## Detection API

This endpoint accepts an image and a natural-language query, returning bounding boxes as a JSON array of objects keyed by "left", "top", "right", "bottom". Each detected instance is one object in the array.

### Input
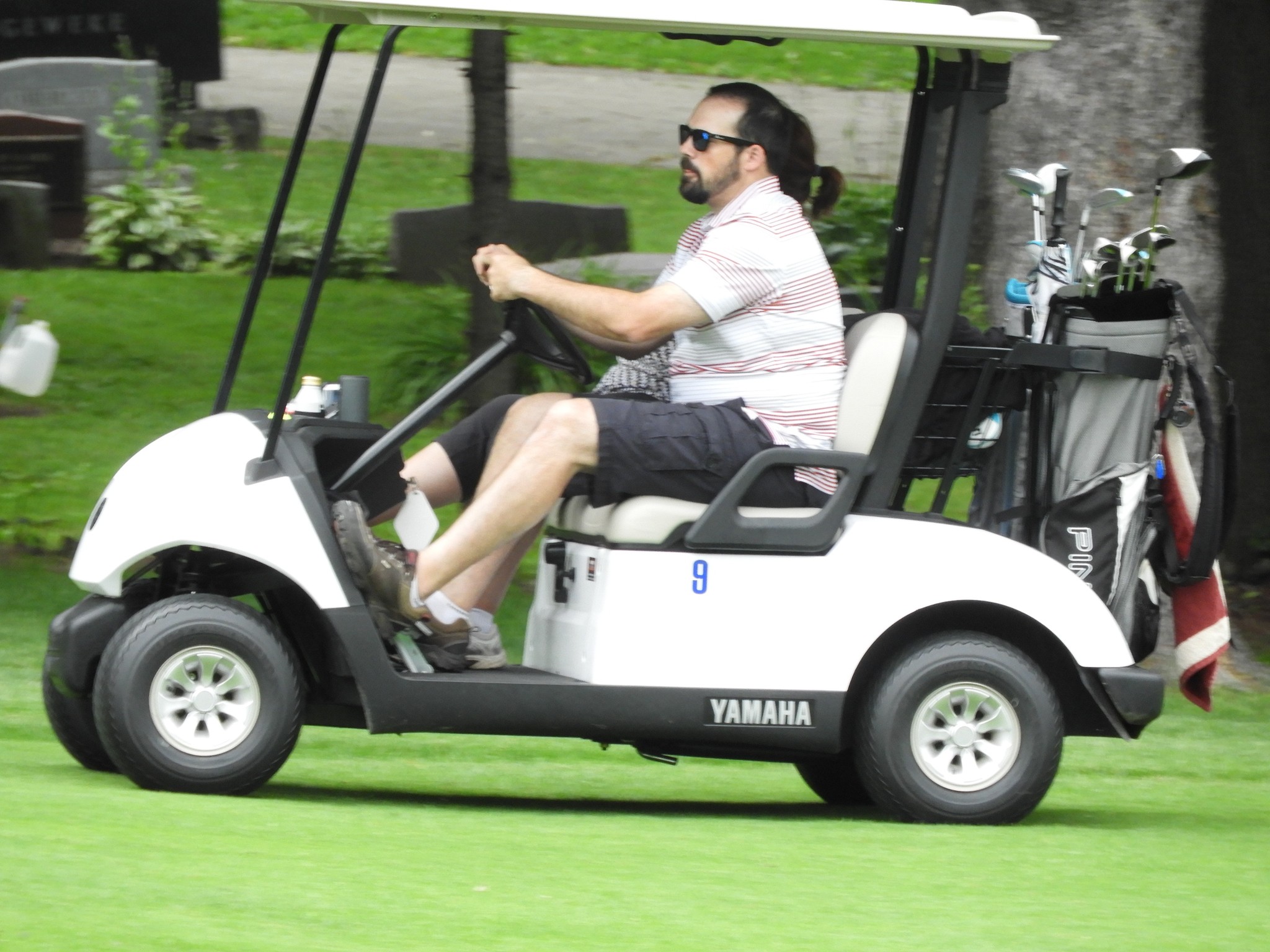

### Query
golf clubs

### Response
[{"left": 1001, "top": 148, "right": 1212, "bottom": 294}]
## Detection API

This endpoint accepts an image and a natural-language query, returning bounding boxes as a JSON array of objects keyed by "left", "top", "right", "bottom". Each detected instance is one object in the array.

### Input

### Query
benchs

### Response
[{"left": 542, "top": 306, "right": 920, "bottom": 559}]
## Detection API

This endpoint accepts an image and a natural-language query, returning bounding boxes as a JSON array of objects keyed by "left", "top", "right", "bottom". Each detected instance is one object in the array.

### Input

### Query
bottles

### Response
[
  {"left": 294, "top": 376, "right": 324, "bottom": 419},
  {"left": 0, "top": 320, "right": 60, "bottom": 398}
]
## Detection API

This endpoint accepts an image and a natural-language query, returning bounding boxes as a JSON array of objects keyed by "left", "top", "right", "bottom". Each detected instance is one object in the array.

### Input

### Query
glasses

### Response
[{"left": 679, "top": 123, "right": 763, "bottom": 153}]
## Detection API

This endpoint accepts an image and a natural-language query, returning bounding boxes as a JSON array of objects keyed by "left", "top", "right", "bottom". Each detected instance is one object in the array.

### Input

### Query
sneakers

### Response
[
  {"left": 332, "top": 500, "right": 430, "bottom": 642},
  {"left": 450, "top": 608, "right": 506, "bottom": 670},
  {"left": 407, "top": 608, "right": 471, "bottom": 673}
]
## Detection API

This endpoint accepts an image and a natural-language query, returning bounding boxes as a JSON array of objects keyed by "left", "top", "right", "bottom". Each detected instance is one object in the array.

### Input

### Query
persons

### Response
[{"left": 331, "top": 81, "right": 848, "bottom": 674}]
